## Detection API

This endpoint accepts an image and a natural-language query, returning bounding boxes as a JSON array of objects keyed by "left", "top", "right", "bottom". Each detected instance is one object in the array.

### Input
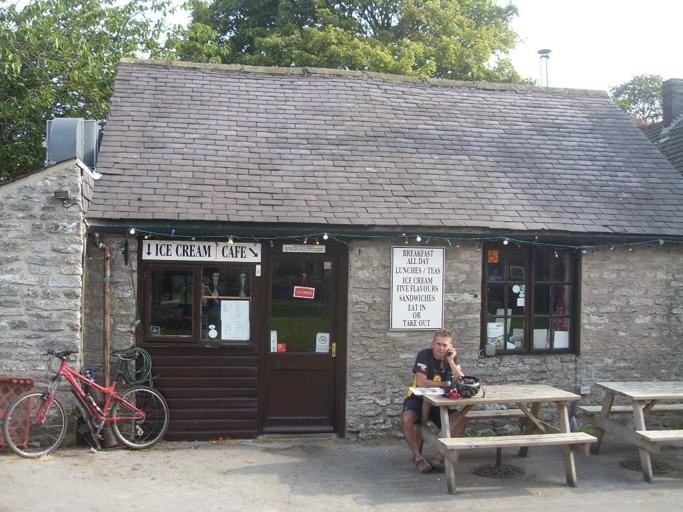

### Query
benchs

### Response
[{"left": 412, "top": 379, "right": 683, "bottom": 495}]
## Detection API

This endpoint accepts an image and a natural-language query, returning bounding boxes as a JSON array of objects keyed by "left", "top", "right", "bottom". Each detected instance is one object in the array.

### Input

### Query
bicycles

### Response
[{"left": 1, "top": 348, "right": 169, "bottom": 459}]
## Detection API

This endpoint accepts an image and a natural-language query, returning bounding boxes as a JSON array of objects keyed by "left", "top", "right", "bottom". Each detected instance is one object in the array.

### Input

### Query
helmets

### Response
[{"left": 456, "top": 375, "right": 481, "bottom": 397}]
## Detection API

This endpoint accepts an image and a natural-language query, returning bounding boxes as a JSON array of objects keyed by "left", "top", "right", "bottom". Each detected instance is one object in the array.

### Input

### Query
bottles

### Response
[{"left": 446, "top": 373, "right": 454, "bottom": 393}]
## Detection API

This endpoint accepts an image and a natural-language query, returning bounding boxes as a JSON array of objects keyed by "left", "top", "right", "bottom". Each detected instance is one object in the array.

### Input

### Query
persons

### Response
[
  {"left": 401, "top": 330, "right": 467, "bottom": 474},
  {"left": 201, "top": 280, "right": 218, "bottom": 338},
  {"left": 157, "top": 292, "right": 170, "bottom": 311}
]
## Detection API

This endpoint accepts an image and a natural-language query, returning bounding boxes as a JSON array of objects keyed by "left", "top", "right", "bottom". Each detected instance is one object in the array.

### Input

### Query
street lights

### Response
[{"left": 537, "top": 48, "right": 551, "bottom": 87}]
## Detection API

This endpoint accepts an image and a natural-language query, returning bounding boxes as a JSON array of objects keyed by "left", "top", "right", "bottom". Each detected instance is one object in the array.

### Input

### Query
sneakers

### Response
[{"left": 415, "top": 454, "right": 444, "bottom": 474}]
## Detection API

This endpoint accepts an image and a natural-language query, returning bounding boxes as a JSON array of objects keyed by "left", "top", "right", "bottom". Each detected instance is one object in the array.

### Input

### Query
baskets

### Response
[{"left": 0, "top": 375, "right": 36, "bottom": 447}]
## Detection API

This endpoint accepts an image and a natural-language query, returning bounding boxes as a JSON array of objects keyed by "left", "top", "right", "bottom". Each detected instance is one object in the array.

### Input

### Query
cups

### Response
[{"left": 484, "top": 343, "right": 495, "bottom": 356}]
